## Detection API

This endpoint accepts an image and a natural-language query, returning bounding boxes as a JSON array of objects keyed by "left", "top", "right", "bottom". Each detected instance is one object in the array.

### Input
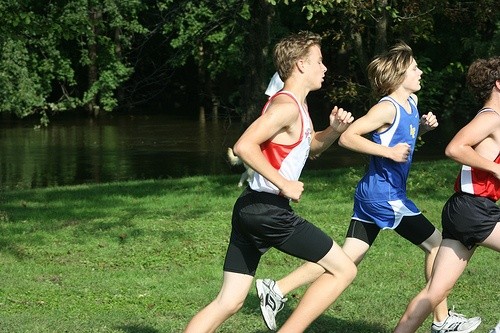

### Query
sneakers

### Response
[
  {"left": 431, "top": 310, "right": 482, "bottom": 333},
  {"left": 256, "top": 279, "right": 288, "bottom": 331}
]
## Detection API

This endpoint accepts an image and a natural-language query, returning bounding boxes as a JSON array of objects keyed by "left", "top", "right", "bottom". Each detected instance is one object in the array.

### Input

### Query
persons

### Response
[
  {"left": 184, "top": 30, "right": 355, "bottom": 333},
  {"left": 392, "top": 57, "right": 500, "bottom": 333},
  {"left": 255, "top": 40, "right": 482, "bottom": 333}
]
